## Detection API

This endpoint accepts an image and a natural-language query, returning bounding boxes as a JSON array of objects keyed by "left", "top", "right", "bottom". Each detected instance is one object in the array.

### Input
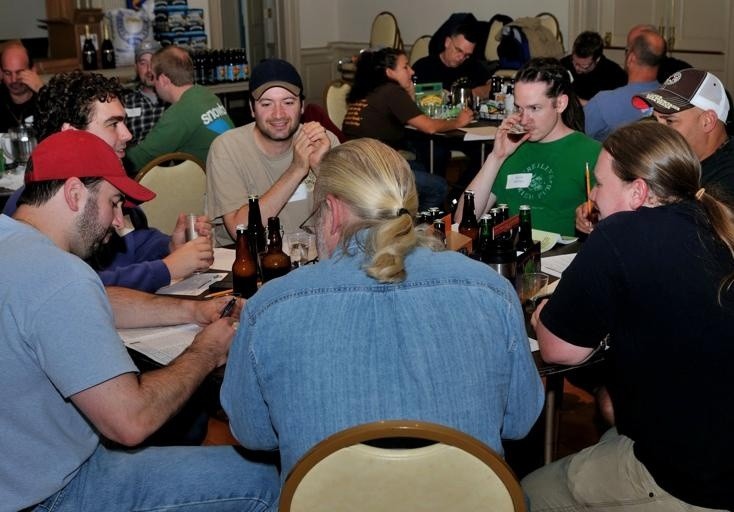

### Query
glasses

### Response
[
  {"left": 298, "top": 205, "right": 323, "bottom": 235},
  {"left": 571, "top": 60, "right": 594, "bottom": 71},
  {"left": 449, "top": 36, "right": 473, "bottom": 61}
]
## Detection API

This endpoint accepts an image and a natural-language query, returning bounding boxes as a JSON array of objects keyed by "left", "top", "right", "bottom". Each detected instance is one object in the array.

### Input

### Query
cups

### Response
[
  {"left": 522, "top": 273, "right": 549, "bottom": 305},
  {"left": 507, "top": 113, "right": 536, "bottom": 143},
  {"left": 286, "top": 233, "right": 311, "bottom": 270}
]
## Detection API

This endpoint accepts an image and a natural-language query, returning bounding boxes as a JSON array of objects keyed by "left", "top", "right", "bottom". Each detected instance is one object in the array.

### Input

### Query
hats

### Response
[
  {"left": 22, "top": 129, "right": 157, "bottom": 208},
  {"left": 248, "top": 57, "right": 304, "bottom": 102},
  {"left": 630, "top": 68, "right": 729, "bottom": 127},
  {"left": 133, "top": 37, "right": 161, "bottom": 64}
]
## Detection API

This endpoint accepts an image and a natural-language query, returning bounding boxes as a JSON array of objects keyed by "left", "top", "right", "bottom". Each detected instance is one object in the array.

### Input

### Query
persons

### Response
[
  {"left": 0, "top": 130, "right": 280, "bottom": 511},
  {"left": 631, "top": 68, "right": 734, "bottom": 216},
  {"left": 519, "top": 121, "right": 734, "bottom": 512},
  {"left": 124, "top": 47, "right": 236, "bottom": 172},
  {"left": 118, "top": 40, "right": 172, "bottom": 152},
  {"left": 454, "top": 56, "right": 603, "bottom": 239},
  {"left": 0, "top": 39, "right": 54, "bottom": 131},
  {"left": 411, "top": 24, "right": 496, "bottom": 105},
  {"left": 625, "top": 25, "right": 694, "bottom": 83},
  {"left": 221, "top": 139, "right": 545, "bottom": 504},
  {"left": 1, "top": 71, "right": 215, "bottom": 288},
  {"left": 559, "top": 31, "right": 628, "bottom": 106},
  {"left": 342, "top": 47, "right": 474, "bottom": 206},
  {"left": 584, "top": 30, "right": 694, "bottom": 153},
  {"left": 205, "top": 58, "right": 341, "bottom": 252}
]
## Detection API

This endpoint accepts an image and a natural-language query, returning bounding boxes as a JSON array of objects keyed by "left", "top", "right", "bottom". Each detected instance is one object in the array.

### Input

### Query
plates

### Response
[{"left": 479, "top": 110, "right": 507, "bottom": 120}]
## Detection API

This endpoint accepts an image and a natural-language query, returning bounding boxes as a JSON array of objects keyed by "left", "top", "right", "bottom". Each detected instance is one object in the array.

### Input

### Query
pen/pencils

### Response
[
  {"left": 205, "top": 282, "right": 263, "bottom": 298},
  {"left": 465, "top": 99, "right": 467, "bottom": 113},
  {"left": 586, "top": 162, "right": 591, "bottom": 214},
  {"left": 219, "top": 297, "right": 237, "bottom": 319}
]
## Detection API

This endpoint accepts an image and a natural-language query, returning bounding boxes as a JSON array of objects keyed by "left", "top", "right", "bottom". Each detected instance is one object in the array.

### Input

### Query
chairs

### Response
[
  {"left": 278, "top": 421, "right": 525, "bottom": 507},
  {"left": 130, "top": 152, "right": 212, "bottom": 244}
]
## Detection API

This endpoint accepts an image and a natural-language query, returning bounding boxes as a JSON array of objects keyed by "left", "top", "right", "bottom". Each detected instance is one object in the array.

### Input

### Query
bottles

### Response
[
  {"left": 232, "top": 223, "right": 257, "bottom": 299},
  {"left": 506, "top": 84, "right": 512, "bottom": 94},
  {"left": 261, "top": 217, "right": 291, "bottom": 284},
  {"left": 82, "top": 25, "right": 97, "bottom": 71},
  {"left": 187, "top": 48, "right": 249, "bottom": 86},
  {"left": 476, "top": 213, "right": 496, "bottom": 264},
  {"left": 245, "top": 194, "right": 265, "bottom": 262},
  {"left": 432, "top": 217, "right": 447, "bottom": 248},
  {"left": 101, "top": 23, "right": 116, "bottom": 69},
  {"left": 515, "top": 204, "right": 535, "bottom": 258},
  {"left": 458, "top": 190, "right": 479, "bottom": 244},
  {"left": 498, "top": 203, "right": 513, "bottom": 260},
  {"left": 489, "top": 75, "right": 497, "bottom": 100},
  {"left": 489, "top": 208, "right": 505, "bottom": 262}
]
